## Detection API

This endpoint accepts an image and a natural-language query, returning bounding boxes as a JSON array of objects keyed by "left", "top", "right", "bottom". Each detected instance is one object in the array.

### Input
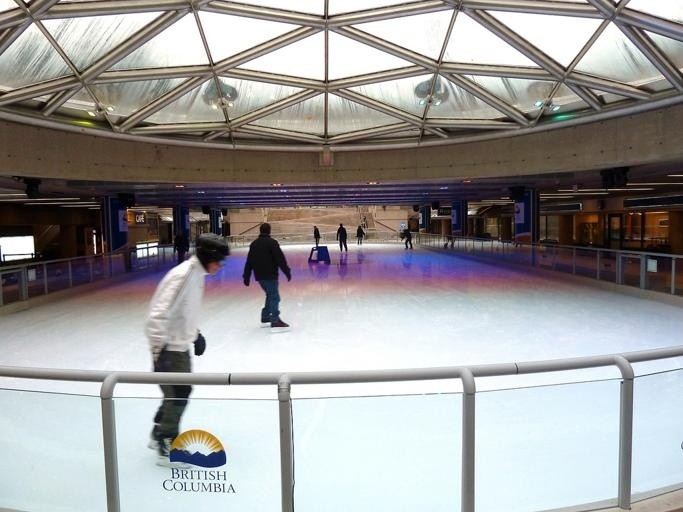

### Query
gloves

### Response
[
  {"left": 194, "top": 334, "right": 206, "bottom": 356},
  {"left": 285, "top": 273, "right": 292, "bottom": 282},
  {"left": 244, "top": 278, "right": 250, "bottom": 286}
]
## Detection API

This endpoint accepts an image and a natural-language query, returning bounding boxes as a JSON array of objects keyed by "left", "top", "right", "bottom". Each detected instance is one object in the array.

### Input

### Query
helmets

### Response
[{"left": 195, "top": 232, "right": 230, "bottom": 263}]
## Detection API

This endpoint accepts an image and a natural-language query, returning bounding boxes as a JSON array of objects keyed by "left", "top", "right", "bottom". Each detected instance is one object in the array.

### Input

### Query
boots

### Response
[
  {"left": 151, "top": 416, "right": 173, "bottom": 457},
  {"left": 271, "top": 319, "right": 289, "bottom": 327},
  {"left": 261, "top": 316, "right": 273, "bottom": 322}
]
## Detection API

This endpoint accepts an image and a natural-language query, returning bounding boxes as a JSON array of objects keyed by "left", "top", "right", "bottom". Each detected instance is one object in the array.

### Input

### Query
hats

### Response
[{"left": 261, "top": 223, "right": 271, "bottom": 234}]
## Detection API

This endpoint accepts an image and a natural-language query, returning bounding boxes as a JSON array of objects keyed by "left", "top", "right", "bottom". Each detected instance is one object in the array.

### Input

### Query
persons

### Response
[
  {"left": 336, "top": 223, "right": 347, "bottom": 252},
  {"left": 144, "top": 233, "right": 229, "bottom": 469},
  {"left": 401, "top": 229, "right": 412, "bottom": 249},
  {"left": 314, "top": 226, "right": 320, "bottom": 246},
  {"left": 174, "top": 229, "right": 189, "bottom": 264},
  {"left": 357, "top": 226, "right": 365, "bottom": 245},
  {"left": 243, "top": 223, "right": 291, "bottom": 327}
]
